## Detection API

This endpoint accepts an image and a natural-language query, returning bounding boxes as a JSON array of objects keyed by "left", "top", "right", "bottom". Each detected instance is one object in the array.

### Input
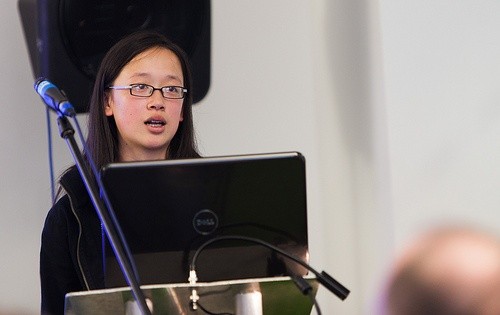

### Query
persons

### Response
[{"left": 40, "top": 31, "right": 310, "bottom": 315}]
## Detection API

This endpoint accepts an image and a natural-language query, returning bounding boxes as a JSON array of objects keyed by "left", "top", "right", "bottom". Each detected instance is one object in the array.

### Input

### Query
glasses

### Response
[{"left": 108, "top": 84, "right": 188, "bottom": 99}]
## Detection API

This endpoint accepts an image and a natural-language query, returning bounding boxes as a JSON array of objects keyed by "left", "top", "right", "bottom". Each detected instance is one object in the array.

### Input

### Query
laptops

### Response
[{"left": 98, "top": 151, "right": 310, "bottom": 288}]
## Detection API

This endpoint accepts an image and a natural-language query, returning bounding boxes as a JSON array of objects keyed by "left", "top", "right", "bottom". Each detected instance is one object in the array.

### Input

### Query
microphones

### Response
[
  {"left": 188, "top": 234, "right": 351, "bottom": 301},
  {"left": 293, "top": 275, "right": 321, "bottom": 315},
  {"left": 33, "top": 77, "right": 76, "bottom": 118}
]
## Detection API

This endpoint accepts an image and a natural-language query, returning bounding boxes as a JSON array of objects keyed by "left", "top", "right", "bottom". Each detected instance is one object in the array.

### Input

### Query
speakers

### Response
[{"left": 17, "top": 0, "right": 211, "bottom": 113}]
